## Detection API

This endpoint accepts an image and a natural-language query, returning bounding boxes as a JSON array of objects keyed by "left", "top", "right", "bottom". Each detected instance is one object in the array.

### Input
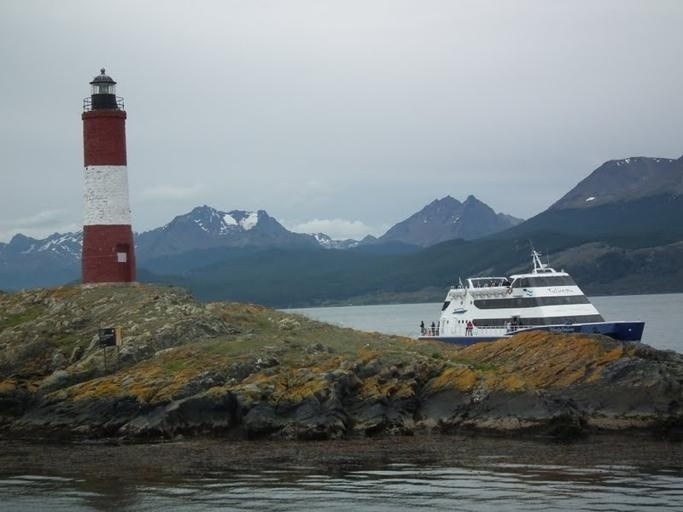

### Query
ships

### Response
[{"left": 418, "top": 234, "right": 645, "bottom": 347}]
